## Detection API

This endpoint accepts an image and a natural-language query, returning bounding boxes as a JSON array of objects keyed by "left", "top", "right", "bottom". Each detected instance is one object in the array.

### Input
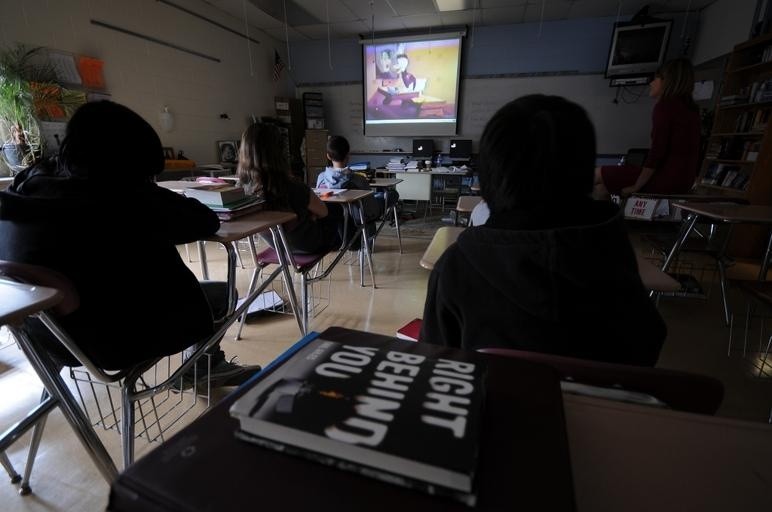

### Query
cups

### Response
[{"left": 425, "top": 161, "right": 431, "bottom": 171}]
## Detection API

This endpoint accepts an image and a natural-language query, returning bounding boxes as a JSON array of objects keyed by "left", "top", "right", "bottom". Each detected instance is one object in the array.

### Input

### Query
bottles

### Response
[
  {"left": 435, "top": 154, "right": 443, "bottom": 167},
  {"left": 440, "top": 217, "right": 454, "bottom": 224}
]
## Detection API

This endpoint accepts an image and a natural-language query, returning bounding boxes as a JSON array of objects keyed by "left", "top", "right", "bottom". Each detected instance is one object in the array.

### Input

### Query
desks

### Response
[
  {"left": 1, "top": 278, "right": 120, "bottom": 498},
  {"left": 98, "top": 323, "right": 770, "bottom": 512},
  {"left": 152, "top": 153, "right": 771, "bottom": 388}
]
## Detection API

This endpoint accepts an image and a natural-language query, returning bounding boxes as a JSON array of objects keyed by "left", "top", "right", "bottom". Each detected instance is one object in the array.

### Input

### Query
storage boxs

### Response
[{"left": 273, "top": 90, "right": 329, "bottom": 186}]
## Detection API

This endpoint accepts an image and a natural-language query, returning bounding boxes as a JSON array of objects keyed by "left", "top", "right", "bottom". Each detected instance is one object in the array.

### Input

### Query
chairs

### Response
[
  {"left": 468, "top": 337, "right": 728, "bottom": 421},
  {"left": 0, "top": 190, "right": 243, "bottom": 496}
]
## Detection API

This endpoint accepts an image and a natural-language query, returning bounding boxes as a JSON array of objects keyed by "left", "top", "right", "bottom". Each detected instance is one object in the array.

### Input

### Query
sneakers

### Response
[{"left": 169, "top": 355, "right": 261, "bottom": 394}]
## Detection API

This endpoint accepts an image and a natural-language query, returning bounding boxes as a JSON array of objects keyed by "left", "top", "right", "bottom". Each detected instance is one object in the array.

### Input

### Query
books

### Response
[
  {"left": 385, "top": 158, "right": 423, "bottom": 172},
  {"left": 229, "top": 338, "right": 489, "bottom": 508},
  {"left": 702, "top": 44, "right": 772, "bottom": 191},
  {"left": 155, "top": 180, "right": 267, "bottom": 222}
]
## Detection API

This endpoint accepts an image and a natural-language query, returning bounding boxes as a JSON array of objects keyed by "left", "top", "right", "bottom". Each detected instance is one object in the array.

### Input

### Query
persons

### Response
[
  {"left": 7, "top": 99, "right": 262, "bottom": 394},
  {"left": 590, "top": 58, "right": 705, "bottom": 200},
  {"left": 419, "top": 94, "right": 726, "bottom": 417},
  {"left": 234, "top": 122, "right": 361, "bottom": 256},
  {"left": 315, "top": 136, "right": 399, "bottom": 249}
]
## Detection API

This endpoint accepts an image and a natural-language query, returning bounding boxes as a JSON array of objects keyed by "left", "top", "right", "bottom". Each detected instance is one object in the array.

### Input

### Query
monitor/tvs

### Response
[{"left": 604, "top": 19, "right": 674, "bottom": 78}]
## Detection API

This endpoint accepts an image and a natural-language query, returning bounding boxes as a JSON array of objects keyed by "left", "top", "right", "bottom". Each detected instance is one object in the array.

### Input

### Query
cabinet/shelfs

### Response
[
  {"left": 38, "top": 82, "right": 112, "bottom": 131},
  {"left": 674, "top": 31, "right": 772, "bottom": 264}
]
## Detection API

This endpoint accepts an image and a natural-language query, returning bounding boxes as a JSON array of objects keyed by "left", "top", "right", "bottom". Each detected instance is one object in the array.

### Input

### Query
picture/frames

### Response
[{"left": 214, "top": 139, "right": 239, "bottom": 165}]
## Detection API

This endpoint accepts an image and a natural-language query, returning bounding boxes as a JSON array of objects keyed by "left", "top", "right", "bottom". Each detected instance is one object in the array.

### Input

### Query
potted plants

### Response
[{"left": 1, "top": 37, "right": 100, "bottom": 179}]
